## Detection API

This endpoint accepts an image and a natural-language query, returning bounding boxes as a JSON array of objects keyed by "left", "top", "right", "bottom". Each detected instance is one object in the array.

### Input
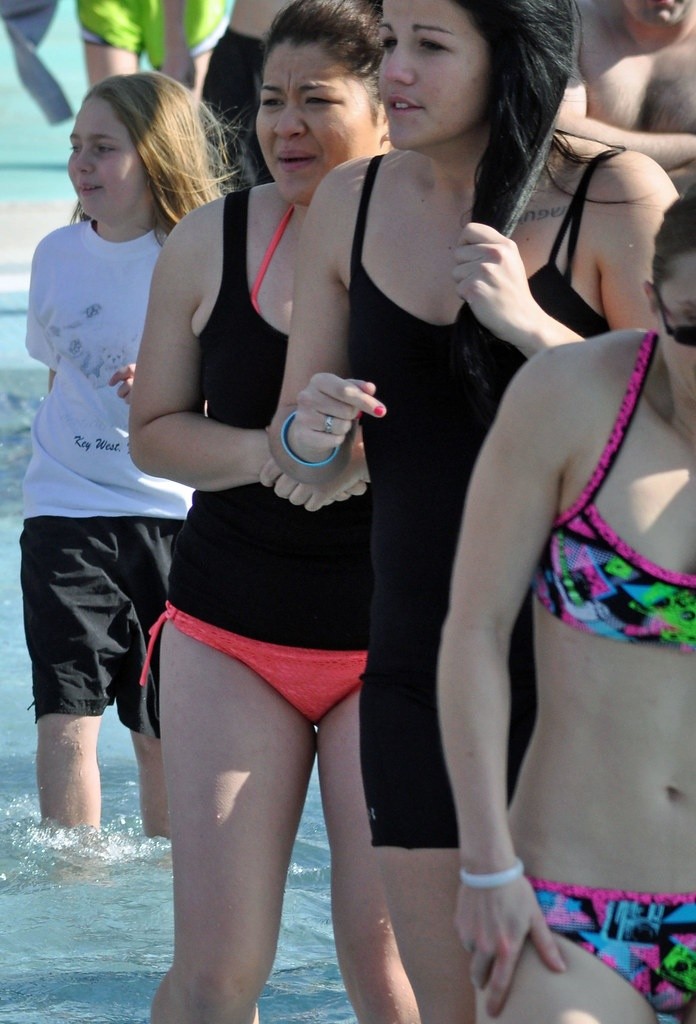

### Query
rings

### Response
[{"left": 324, "top": 415, "right": 334, "bottom": 433}]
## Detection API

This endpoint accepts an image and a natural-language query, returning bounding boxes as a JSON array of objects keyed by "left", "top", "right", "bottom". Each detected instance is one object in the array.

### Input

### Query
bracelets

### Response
[
  {"left": 458, "top": 859, "right": 524, "bottom": 888},
  {"left": 283, "top": 411, "right": 341, "bottom": 468}
]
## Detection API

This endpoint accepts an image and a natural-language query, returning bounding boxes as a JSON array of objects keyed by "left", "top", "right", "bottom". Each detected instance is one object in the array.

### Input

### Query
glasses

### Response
[{"left": 653, "top": 284, "right": 696, "bottom": 347}]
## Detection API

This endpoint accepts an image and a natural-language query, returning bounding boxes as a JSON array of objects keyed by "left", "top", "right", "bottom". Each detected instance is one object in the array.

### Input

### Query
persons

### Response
[{"left": 0, "top": 0, "right": 696, "bottom": 1024}]
